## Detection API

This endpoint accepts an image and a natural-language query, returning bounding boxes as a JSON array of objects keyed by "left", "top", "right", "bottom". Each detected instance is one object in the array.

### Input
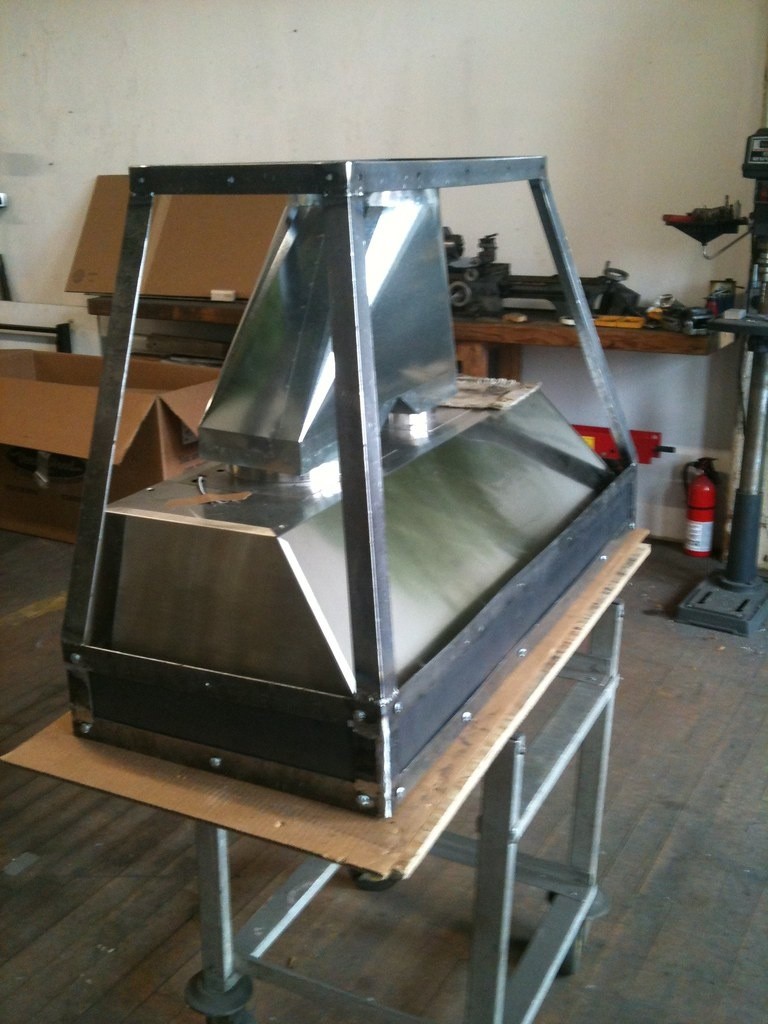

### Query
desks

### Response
[
  {"left": 88, "top": 295, "right": 746, "bottom": 381},
  {"left": 0, "top": 525, "right": 651, "bottom": 1023}
]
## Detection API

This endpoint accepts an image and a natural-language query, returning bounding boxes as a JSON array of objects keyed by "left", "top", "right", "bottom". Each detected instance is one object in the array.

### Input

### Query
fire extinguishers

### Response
[{"left": 680, "top": 457, "right": 720, "bottom": 557}]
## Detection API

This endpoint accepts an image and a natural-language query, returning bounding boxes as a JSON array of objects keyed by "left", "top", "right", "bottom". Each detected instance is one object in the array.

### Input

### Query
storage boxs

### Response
[
  {"left": 64, "top": 173, "right": 290, "bottom": 299},
  {"left": 0, "top": 348, "right": 225, "bottom": 542}
]
